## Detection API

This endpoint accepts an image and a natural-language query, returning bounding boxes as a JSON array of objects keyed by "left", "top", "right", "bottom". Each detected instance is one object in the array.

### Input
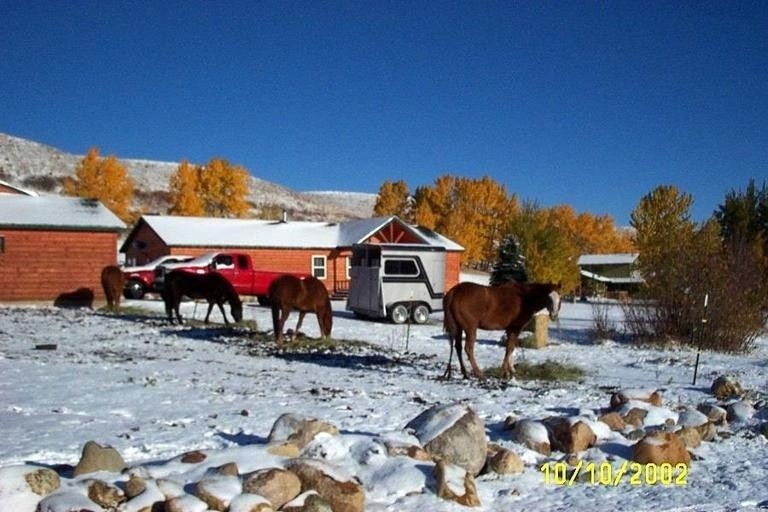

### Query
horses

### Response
[
  {"left": 159, "top": 269, "right": 244, "bottom": 327},
  {"left": 270, "top": 274, "right": 333, "bottom": 345},
  {"left": 441, "top": 279, "right": 563, "bottom": 381},
  {"left": 101, "top": 266, "right": 127, "bottom": 310}
]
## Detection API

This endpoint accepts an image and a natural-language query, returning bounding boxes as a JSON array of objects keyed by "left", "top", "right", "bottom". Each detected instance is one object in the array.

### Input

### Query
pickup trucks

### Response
[
  {"left": 119, "top": 252, "right": 197, "bottom": 301},
  {"left": 154, "top": 247, "right": 313, "bottom": 308}
]
